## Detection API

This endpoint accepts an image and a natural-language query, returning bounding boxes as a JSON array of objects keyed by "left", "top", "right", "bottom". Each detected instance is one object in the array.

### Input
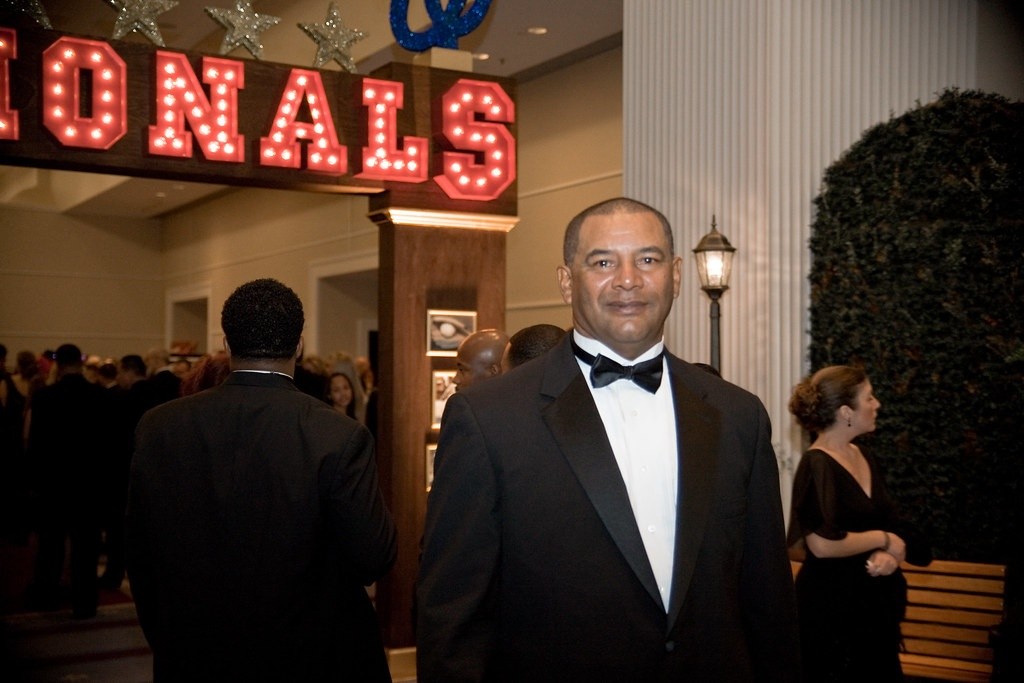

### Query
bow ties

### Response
[{"left": 570, "top": 329, "right": 665, "bottom": 395}]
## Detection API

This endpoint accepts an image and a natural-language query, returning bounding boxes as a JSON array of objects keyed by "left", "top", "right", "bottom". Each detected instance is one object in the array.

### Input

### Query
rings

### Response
[{"left": 878, "top": 568, "right": 882, "bottom": 572}]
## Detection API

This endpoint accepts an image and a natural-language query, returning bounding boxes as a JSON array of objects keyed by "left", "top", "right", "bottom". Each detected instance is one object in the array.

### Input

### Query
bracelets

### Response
[{"left": 882, "top": 530, "right": 891, "bottom": 552}]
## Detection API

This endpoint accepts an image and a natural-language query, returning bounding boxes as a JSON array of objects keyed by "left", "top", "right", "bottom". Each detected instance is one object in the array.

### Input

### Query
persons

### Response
[
  {"left": 0, "top": 341, "right": 377, "bottom": 619},
  {"left": 504, "top": 324, "right": 566, "bottom": 375},
  {"left": 415, "top": 198, "right": 807, "bottom": 683},
  {"left": 451, "top": 328, "right": 510, "bottom": 392},
  {"left": 125, "top": 278, "right": 397, "bottom": 683},
  {"left": 782, "top": 365, "right": 910, "bottom": 683}
]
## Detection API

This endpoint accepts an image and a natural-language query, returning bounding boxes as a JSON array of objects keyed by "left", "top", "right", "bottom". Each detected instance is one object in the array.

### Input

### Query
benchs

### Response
[{"left": 784, "top": 555, "right": 1006, "bottom": 682}]
